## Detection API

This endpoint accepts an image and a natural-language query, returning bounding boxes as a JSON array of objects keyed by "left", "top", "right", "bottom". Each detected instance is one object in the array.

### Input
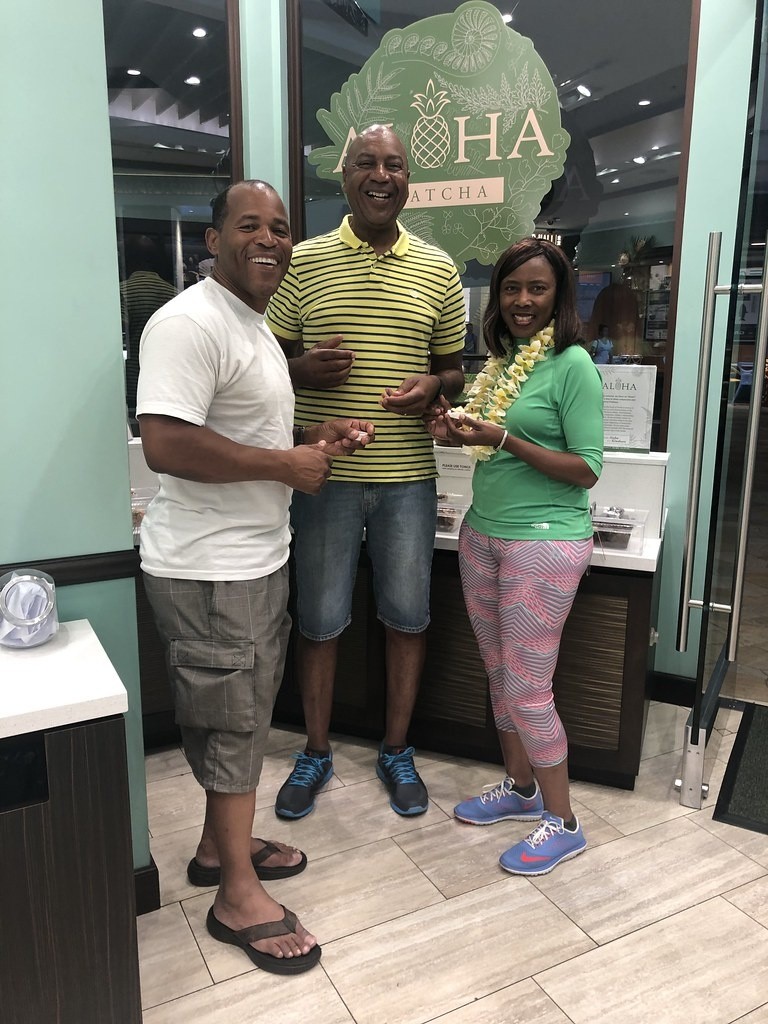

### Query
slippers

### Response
[
  {"left": 205, "top": 903, "right": 322, "bottom": 975},
  {"left": 188, "top": 838, "right": 308, "bottom": 887}
]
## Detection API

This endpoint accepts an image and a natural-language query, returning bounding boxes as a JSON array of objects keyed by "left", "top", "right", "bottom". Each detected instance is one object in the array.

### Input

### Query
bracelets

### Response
[
  {"left": 493, "top": 429, "right": 508, "bottom": 452},
  {"left": 293, "top": 427, "right": 305, "bottom": 447},
  {"left": 432, "top": 378, "right": 443, "bottom": 404}
]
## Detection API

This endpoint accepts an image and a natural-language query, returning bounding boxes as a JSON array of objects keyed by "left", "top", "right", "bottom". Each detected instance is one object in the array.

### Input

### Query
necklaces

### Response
[{"left": 448, "top": 317, "right": 554, "bottom": 463}]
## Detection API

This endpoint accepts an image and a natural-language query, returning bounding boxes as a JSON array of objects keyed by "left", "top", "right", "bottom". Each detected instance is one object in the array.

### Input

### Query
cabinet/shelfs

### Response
[
  {"left": 267, "top": 539, "right": 661, "bottom": 790},
  {"left": 0, "top": 618, "right": 144, "bottom": 1024}
]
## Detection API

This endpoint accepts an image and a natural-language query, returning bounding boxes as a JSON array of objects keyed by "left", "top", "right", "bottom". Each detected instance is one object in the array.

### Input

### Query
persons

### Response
[
  {"left": 135, "top": 179, "right": 375, "bottom": 975},
  {"left": 589, "top": 325, "right": 614, "bottom": 364},
  {"left": 586, "top": 283, "right": 658, "bottom": 365},
  {"left": 428, "top": 237, "right": 604, "bottom": 876},
  {"left": 263, "top": 124, "right": 466, "bottom": 820},
  {"left": 463, "top": 323, "right": 477, "bottom": 373},
  {"left": 120, "top": 235, "right": 181, "bottom": 437}
]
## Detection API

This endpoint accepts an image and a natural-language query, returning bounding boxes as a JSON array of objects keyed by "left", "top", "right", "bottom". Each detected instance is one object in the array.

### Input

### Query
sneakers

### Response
[
  {"left": 498, "top": 810, "right": 587, "bottom": 876},
  {"left": 275, "top": 742, "right": 334, "bottom": 817},
  {"left": 376, "top": 738, "right": 429, "bottom": 814},
  {"left": 454, "top": 766, "right": 544, "bottom": 825}
]
